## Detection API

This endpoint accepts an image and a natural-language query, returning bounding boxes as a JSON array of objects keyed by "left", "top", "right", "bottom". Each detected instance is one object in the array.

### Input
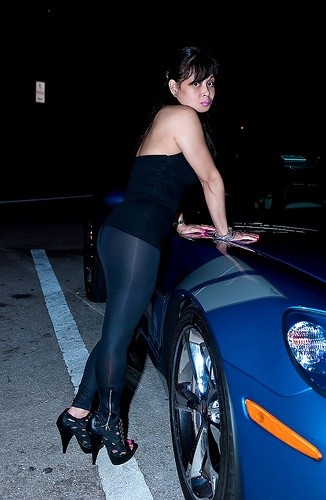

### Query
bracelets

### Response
[
  {"left": 175, "top": 219, "right": 184, "bottom": 226},
  {"left": 215, "top": 227, "right": 236, "bottom": 241}
]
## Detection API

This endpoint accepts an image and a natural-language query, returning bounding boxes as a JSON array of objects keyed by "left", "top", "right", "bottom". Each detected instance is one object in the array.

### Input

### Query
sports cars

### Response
[{"left": 81, "top": 190, "right": 324, "bottom": 499}]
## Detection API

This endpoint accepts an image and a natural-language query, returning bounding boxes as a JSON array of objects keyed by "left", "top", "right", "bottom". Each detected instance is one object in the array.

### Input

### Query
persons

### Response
[{"left": 57, "top": 50, "right": 259, "bottom": 472}]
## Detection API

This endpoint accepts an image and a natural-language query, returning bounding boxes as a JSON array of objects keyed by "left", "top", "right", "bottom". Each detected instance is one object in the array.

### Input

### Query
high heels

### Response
[{"left": 56, "top": 408, "right": 138, "bottom": 465}]
